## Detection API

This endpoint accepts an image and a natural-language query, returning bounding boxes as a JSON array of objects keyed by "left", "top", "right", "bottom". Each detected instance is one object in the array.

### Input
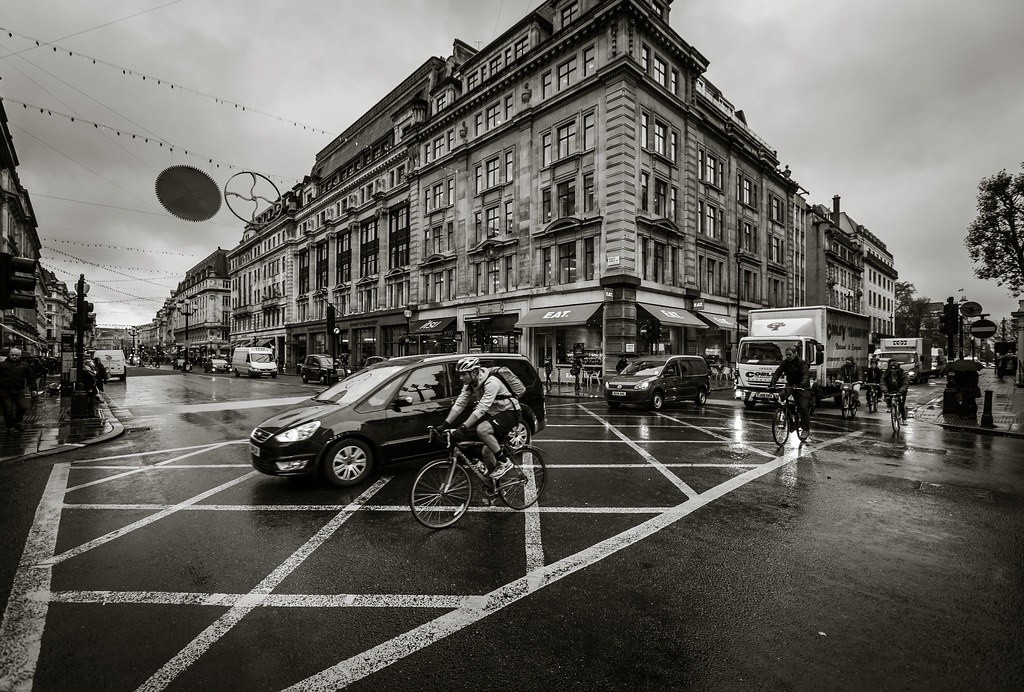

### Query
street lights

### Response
[
  {"left": 404, "top": 305, "right": 412, "bottom": 355},
  {"left": 128, "top": 295, "right": 198, "bottom": 371},
  {"left": 733, "top": 245, "right": 744, "bottom": 349},
  {"left": 72, "top": 274, "right": 90, "bottom": 419},
  {"left": 253, "top": 329, "right": 257, "bottom": 347}
]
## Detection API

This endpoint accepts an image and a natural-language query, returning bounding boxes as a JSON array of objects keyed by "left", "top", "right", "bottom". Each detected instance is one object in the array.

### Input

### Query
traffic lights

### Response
[
  {"left": 971, "top": 319, "right": 997, "bottom": 339},
  {"left": 0, "top": 253, "right": 37, "bottom": 310},
  {"left": 83, "top": 301, "right": 94, "bottom": 331},
  {"left": 940, "top": 304, "right": 959, "bottom": 334}
]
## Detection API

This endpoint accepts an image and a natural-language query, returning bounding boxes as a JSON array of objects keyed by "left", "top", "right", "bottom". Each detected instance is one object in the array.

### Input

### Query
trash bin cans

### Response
[{"left": 71, "top": 391, "right": 87, "bottom": 417}]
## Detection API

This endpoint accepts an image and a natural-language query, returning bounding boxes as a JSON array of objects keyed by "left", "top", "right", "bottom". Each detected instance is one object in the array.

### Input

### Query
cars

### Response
[
  {"left": 300, "top": 353, "right": 394, "bottom": 386},
  {"left": 172, "top": 359, "right": 233, "bottom": 374}
]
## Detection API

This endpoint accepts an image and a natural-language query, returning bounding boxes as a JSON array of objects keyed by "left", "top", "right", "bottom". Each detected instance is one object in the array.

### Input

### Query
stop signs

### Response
[{"left": 640, "top": 325, "right": 648, "bottom": 335}]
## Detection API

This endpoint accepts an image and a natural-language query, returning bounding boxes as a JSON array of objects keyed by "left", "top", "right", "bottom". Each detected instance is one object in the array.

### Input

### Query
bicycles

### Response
[
  {"left": 409, "top": 426, "right": 548, "bottom": 530},
  {"left": 767, "top": 381, "right": 903, "bottom": 447}
]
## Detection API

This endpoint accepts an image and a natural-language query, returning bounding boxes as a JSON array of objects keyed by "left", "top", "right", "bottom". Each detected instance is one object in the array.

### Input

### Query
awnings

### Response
[
  {"left": 220, "top": 338, "right": 274, "bottom": 349},
  {"left": 638, "top": 303, "right": 710, "bottom": 329},
  {"left": 409, "top": 317, "right": 456, "bottom": 335},
  {"left": 698, "top": 312, "right": 748, "bottom": 329},
  {"left": 513, "top": 303, "right": 603, "bottom": 328}
]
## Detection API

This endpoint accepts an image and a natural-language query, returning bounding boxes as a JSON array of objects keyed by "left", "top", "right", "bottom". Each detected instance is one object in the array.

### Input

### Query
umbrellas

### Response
[
  {"left": 948, "top": 360, "right": 984, "bottom": 371},
  {"left": 618, "top": 352, "right": 637, "bottom": 358},
  {"left": 573, "top": 354, "right": 589, "bottom": 357}
]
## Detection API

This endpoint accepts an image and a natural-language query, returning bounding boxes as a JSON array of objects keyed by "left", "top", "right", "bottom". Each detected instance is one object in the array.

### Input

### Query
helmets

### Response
[{"left": 453, "top": 356, "right": 482, "bottom": 373}]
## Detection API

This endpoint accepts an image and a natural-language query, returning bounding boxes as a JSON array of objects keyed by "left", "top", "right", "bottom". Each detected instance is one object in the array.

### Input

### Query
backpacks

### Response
[{"left": 480, "top": 366, "right": 526, "bottom": 401}]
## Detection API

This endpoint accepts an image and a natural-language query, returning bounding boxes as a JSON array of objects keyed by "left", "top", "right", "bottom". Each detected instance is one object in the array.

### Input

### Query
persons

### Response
[
  {"left": 94, "top": 358, "right": 108, "bottom": 393},
  {"left": 768, "top": 346, "right": 811, "bottom": 439},
  {"left": 616, "top": 357, "right": 628, "bottom": 372},
  {"left": 433, "top": 357, "right": 522, "bottom": 479},
  {"left": 0, "top": 348, "right": 38, "bottom": 433},
  {"left": 865, "top": 358, "right": 882, "bottom": 407},
  {"left": 996, "top": 356, "right": 1007, "bottom": 383},
  {"left": 836, "top": 356, "right": 860, "bottom": 402},
  {"left": 572, "top": 357, "right": 581, "bottom": 391},
  {"left": 957, "top": 370, "right": 979, "bottom": 420},
  {"left": 880, "top": 360, "right": 909, "bottom": 425},
  {"left": 542, "top": 358, "right": 553, "bottom": 386}
]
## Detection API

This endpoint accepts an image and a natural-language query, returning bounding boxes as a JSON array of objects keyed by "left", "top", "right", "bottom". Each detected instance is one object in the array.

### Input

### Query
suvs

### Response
[{"left": 250, "top": 353, "right": 548, "bottom": 486}]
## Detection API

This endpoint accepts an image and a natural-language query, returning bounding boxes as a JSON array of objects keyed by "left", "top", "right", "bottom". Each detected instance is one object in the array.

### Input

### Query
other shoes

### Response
[
  {"left": 855, "top": 400, "right": 861, "bottom": 407},
  {"left": 878, "top": 398, "right": 882, "bottom": 403},
  {"left": 6, "top": 426, "right": 22, "bottom": 434},
  {"left": 799, "top": 429, "right": 810, "bottom": 439},
  {"left": 885, "top": 406, "right": 892, "bottom": 413},
  {"left": 902, "top": 418, "right": 908, "bottom": 426},
  {"left": 866, "top": 402, "right": 869, "bottom": 407}
]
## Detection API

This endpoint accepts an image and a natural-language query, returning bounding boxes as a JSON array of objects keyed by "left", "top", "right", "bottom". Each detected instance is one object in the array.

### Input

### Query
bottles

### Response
[{"left": 472, "top": 457, "right": 488, "bottom": 475}]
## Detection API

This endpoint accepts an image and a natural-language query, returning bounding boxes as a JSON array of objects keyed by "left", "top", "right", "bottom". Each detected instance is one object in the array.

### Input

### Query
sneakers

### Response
[
  {"left": 489, "top": 457, "right": 514, "bottom": 479},
  {"left": 481, "top": 489, "right": 507, "bottom": 505}
]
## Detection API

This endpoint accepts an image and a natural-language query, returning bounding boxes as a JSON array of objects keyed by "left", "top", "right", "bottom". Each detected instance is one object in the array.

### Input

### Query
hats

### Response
[
  {"left": 845, "top": 356, "right": 854, "bottom": 362},
  {"left": 869, "top": 358, "right": 879, "bottom": 365},
  {"left": 887, "top": 359, "right": 899, "bottom": 368}
]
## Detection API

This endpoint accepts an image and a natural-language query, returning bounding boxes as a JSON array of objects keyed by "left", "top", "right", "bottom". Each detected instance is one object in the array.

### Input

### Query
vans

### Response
[
  {"left": 233, "top": 347, "right": 278, "bottom": 379},
  {"left": 931, "top": 348, "right": 946, "bottom": 378},
  {"left": 604, "top": 354, "right": 711, "bottom": 413},
  {"left": 94, "top": 350, "right": 127, "bottom": 382}
]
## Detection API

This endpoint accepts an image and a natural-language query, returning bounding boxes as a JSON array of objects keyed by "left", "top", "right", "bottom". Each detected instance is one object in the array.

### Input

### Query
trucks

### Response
[{"left": 726, "top": 305, "right": 932, "bottom": 412}]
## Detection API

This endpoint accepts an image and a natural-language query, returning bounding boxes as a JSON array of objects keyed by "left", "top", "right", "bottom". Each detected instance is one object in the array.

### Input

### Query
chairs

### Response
[
  {"left": 584, "top": 371, "right": 601, "bottom": 386},
  {"left": 712, "top": 367, "right": 731, "bottom": 380}
]
